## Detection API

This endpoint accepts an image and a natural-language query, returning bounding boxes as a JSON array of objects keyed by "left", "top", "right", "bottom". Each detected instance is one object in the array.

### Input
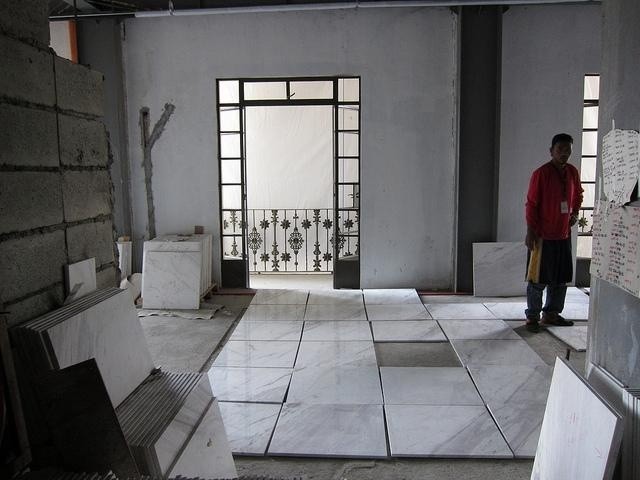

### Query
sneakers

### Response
[
  {"left": 542, "top": 315, "right": 574, "bottom": 327},
  {"left": 526, "top": 316, "right": 539, "bottom": 333}
]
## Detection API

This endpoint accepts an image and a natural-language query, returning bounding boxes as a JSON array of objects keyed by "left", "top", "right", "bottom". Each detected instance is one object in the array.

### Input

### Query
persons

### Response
[{"left": 525, "top": 133, "right": 584, "bottom": 333}]
